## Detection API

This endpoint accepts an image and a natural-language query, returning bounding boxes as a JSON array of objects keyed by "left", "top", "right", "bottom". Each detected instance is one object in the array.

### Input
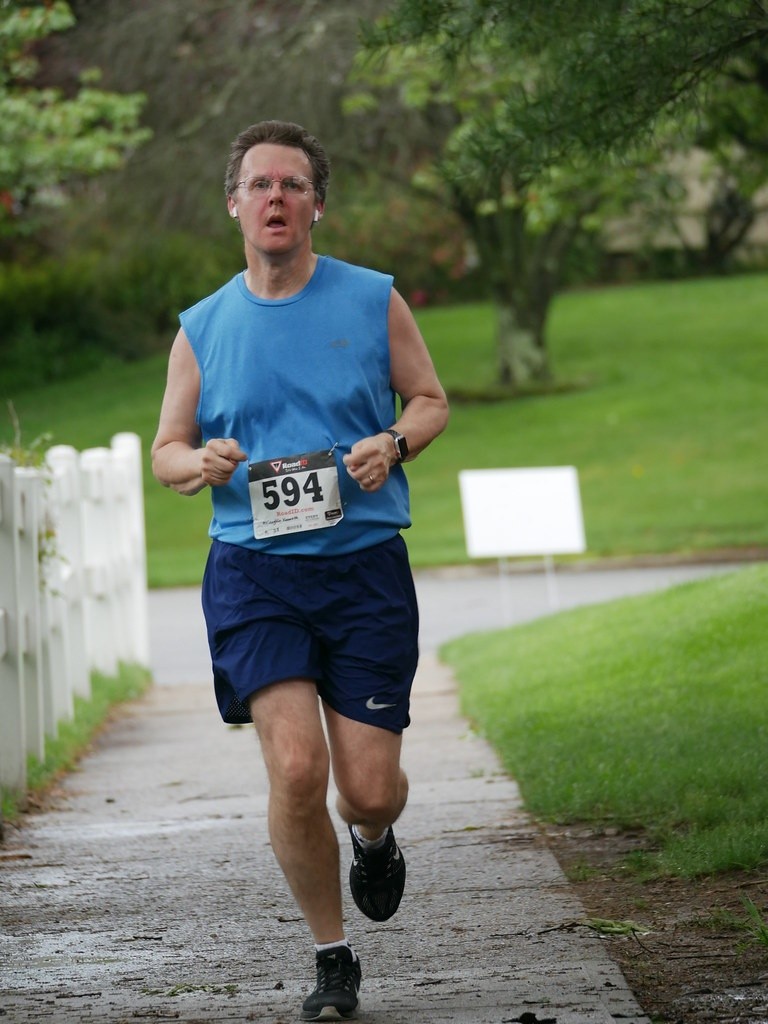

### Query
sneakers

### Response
[
  {"left": 348, "top": 824, "right": 407, "bottom": 922},
  {"left": 299, "top": 944, "right": 363, "bottom": 1020}
]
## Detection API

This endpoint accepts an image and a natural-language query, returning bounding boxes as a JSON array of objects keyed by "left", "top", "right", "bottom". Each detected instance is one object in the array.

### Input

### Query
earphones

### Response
[
  {"left": 233, "top": 207, "right": 238, "bottom": 218},
  {"left": 314, "top": 209, "right": 318, "bottom": 221}
]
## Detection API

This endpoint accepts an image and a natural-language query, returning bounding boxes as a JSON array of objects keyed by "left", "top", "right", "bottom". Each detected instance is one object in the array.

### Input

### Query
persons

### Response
[{"left": 147, "top": 117, "right": 454, "bottom": 1024}]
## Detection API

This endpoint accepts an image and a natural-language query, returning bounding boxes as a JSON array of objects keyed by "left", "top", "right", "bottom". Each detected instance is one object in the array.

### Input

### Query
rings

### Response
[{"left": 368, "top": 474, "right": 375, "bottom": 484}]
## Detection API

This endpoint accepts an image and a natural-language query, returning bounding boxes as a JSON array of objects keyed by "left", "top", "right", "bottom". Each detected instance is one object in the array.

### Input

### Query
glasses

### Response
[{"left": 233, "top": 175, "right": 314, "bottom": 196}]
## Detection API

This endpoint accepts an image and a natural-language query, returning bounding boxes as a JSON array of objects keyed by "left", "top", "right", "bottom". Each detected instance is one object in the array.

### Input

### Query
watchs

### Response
[{"left": 379, "top": 428, "right": 409, "bottom": 466}]
